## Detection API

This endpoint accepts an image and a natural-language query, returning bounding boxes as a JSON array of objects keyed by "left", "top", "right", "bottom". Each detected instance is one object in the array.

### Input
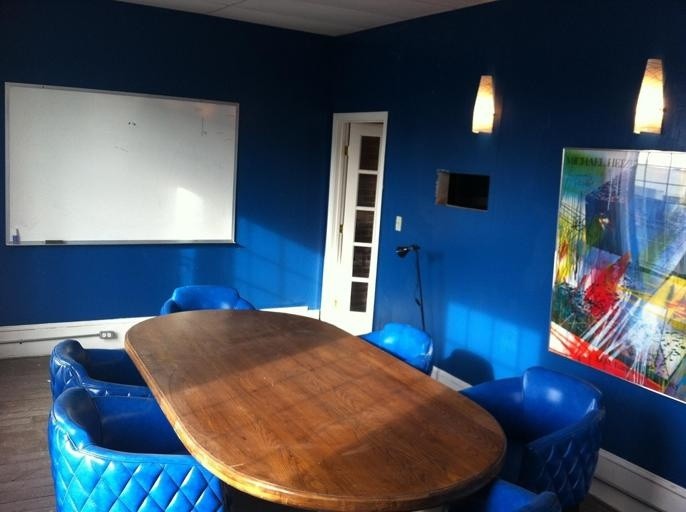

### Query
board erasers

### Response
[{"left": 45, "top": 240, "right": 63, "bottom": 244}]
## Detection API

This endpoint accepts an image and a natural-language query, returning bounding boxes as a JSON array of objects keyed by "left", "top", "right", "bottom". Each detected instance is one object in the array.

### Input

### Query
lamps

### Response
[
  {"left": 631, "top": 57, "right": 668, "bottom": 137},
  {"left": 471, "top": 71, "right": 505, "bottom": 136}
]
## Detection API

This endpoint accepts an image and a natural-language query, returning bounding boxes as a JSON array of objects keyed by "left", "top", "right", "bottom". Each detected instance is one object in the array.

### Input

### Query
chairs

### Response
[
  {"left": 46, "top": 338, "right": 228, "bottom": 512},
  {"left": 457, "top": 368, "right": 608, "bottom": 511},
  {"left": 356, "top": 321, "right": 434, "bottom": 376},
  {"left": 161, "top": 284, "right": 255, "bottom": 314}
]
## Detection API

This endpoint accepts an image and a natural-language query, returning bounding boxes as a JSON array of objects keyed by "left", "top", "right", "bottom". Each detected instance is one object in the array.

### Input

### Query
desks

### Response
[{"left": 124, "top": 310, "right": 507, "bottom": 510}]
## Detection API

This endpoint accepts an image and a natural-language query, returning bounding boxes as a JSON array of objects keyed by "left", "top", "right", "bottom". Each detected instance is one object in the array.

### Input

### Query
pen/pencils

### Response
[{"left": 16, "top": 228, "right": 19, "bottom": 237}]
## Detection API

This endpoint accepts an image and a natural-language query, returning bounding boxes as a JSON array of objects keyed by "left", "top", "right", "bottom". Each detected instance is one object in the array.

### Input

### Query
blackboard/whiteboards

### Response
[{"left": 5, "top": 81, "right": 240, "bottom": 246}]
